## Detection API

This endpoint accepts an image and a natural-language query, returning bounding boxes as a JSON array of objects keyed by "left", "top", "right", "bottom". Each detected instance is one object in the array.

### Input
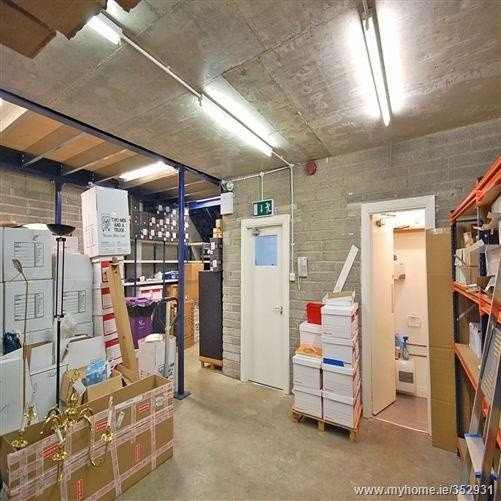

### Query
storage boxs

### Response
[
  {"left": 299, "top": 320, "right": 323, "bottom": 357},
  {"left": 0, "top": 375, "right": 173, "bottom": 501},
  {"left": 321, "top": 390, "right": 363, "bottom": 428},
  {"left": 321, "top": 302, "right": 359, "bottom": 338},
  {"left": 291, "top": 385, "right": 322, "bottom": 419},
  {"left": 184, "top": 301, "right": 195, "bottom": 350},
  {"left": 58, "top": 323, "right": 93, "bottom": 363},
  {"left": 53, "top": 252, "right": 93, "bottom": 326},
  {"left": 210, "top": 219, "right": 223, "bottom": 273},
  {"left": 307, "top": 303, "right": 324, "bottom": 325},
  {"left": 321, "top": 337, "right": 360, "bottom": 368},
  {"left": 449, "top": 155, "right": 501, "bottom": 501},
  {"left": 104, "top": 339, "right": 123, "bottom": 361},
  {"left": 90, "top": 256, "right": 125, "bottom": 281},
  {"left": 167, "top": 286, "right": 180, "bottom": 298},
  {"left": 184, "top": 264, "right": 204, "bottom": 301},
  {"left": 127, "top": 286, "right": 163, "bottom": 301},
  {"left": 322, "top": 363, "right": 361, "bottom": 398},
  {"left": 0, "top": 335, "right": 107, "bottom": 436},
  {"left": 468, "top": 321, "right": 483, "bottom": 358},
  {"left": 456, "top": 266, "right": 481, "bottom": 284},
  {"left": 455, "top": 248, "right": 463, "bottom": 265},
  {"left": 92, "top": 283, "right": 125, "bottom": 315},
  {"left": 14, "top": 328, "right": 57, "bottom": 368},
  {"left": 462, "top": 248, "right": 480, "bottom": 266},
  {"left": 93, "top": 314, "right": 118, "bottom": 342},
  {"left": 142, "top": 203, "right": 190, "bottom": 242},
  {"left": 292, "top": 354, "right": 323, "bottom": 390},
  {"left": 4, "top": 222, "right": 52, "bottom": 282},
  {"left": 138, "top": 333, "right": 176, "bottom": 395},
  {"left": 469, "top": 464, "right": 490, "bottom": 500},
  {"left": 71, "top": 369, "right": 131, "bottom": 405},
  {"left": 3, "top": 280, "right": 55, "bottom": 334},
  {"left": 59, "top": 364, "right": 137, "bottom": 409},
  {"left": 80, "top": 186, "right": 132, "bottom": 258}
]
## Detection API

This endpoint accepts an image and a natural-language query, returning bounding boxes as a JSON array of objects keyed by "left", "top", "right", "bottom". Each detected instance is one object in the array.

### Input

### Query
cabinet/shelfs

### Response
[
  {"left": 124, "top": 240, "right": 210, "bottom": 301},
  {"left": 198, "top": 267, "right": 223, "bottom": 369}
]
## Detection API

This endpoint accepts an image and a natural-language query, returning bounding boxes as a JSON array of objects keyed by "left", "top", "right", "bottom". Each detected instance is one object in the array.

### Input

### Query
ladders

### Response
[{"left": 458, "top": 258, "right": 501, "bottom": 501}]
